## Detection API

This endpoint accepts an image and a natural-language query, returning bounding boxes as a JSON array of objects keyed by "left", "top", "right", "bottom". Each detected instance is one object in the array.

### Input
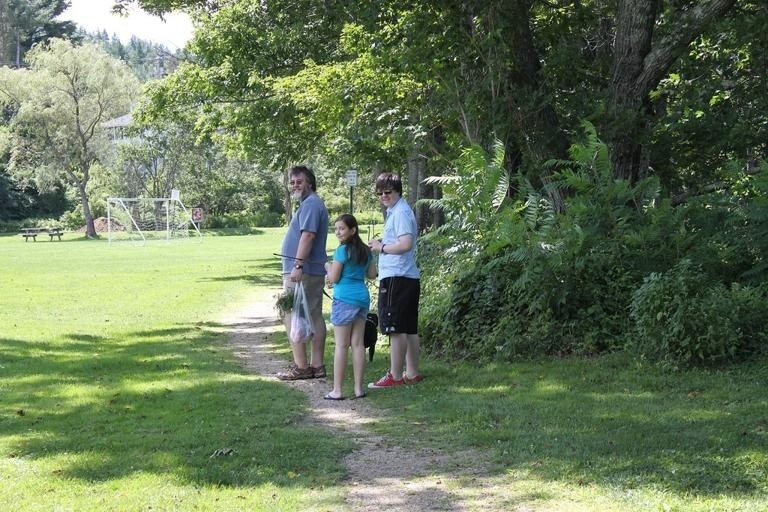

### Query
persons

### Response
[
  {"left": 365, "top": 171, "right": 423, "bottom": 389},
  {"left": 274, "top": 164, "right": 327, "bottom": 381},
  {"left": 323, "top": 213, "right": 376, "bottom": 400}
]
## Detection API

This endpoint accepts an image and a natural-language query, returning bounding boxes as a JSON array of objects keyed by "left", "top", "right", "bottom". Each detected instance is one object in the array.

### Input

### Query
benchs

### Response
[{"left": 22, "top": 233, "right": 63, "bottom": 242}]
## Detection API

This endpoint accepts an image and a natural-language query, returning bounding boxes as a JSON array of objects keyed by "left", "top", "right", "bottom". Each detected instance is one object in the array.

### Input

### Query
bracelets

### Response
[
  {"left": 292, "top": 264, "right": 304, "bottom": 270},
  {"left": 378, "top": 242, "right": 386, "bottom": 254}
]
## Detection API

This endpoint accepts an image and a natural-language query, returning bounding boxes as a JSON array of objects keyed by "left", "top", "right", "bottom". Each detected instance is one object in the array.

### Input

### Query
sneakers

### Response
[
  {"left": 367, "top": 372, "right": 405, "bottom": 389},
  {"left": 401, "top": 373, "right": 424, "bottom": 385},
  {"left": 277, "top": 364, "right": 313, "bottom": 380},
  {"left": 311, "top": 365, "right": 326, "bottom": 379}
]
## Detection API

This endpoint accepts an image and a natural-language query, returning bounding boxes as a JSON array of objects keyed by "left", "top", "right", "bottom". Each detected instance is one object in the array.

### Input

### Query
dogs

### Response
[{"left": 363, "top": 312, "right": 379, "bottom": 362}]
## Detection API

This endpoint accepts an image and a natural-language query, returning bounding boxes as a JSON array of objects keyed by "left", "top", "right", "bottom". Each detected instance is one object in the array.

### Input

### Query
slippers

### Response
[
  {"left": 355, "top": 390, "right": 367, "bottom": 398},
  {"left": 323, "top": 392, "right": 345, "bottom": 400}
]
